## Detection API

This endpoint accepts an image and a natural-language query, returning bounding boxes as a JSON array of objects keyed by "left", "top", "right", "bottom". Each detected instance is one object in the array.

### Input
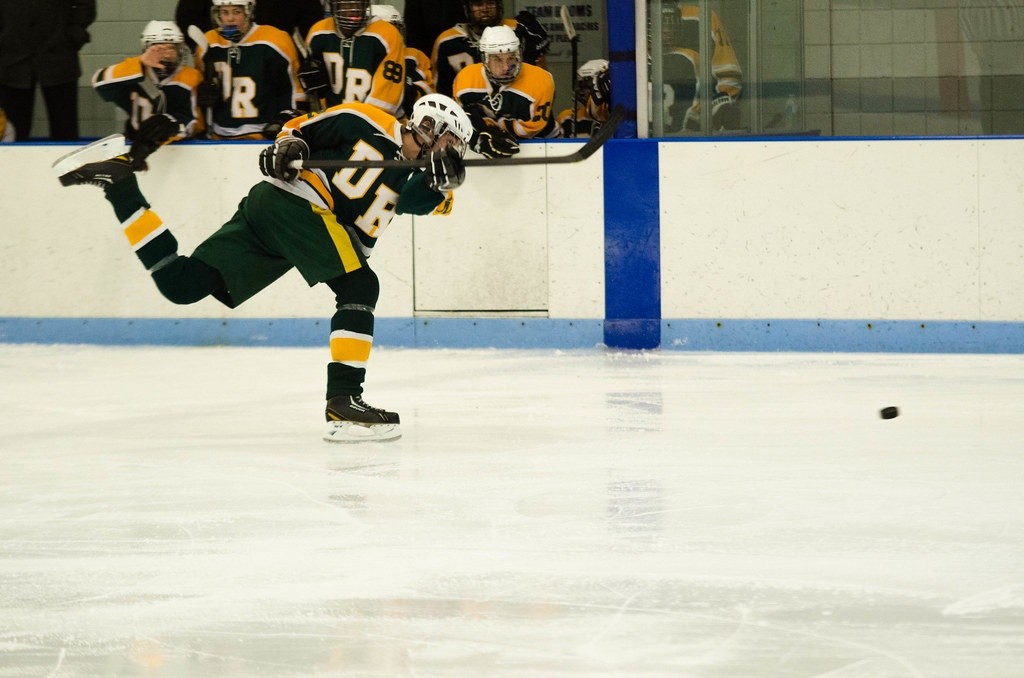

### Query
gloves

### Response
[
  {"left": 262, "top": 109, "right": 308, "bottom": 140},
  {"left": 471, "top": 128, "right": 521, "bottom": 162},
  {"left": 196, "top": 76, "right": 221, "bottom": 109},
  {"left": 259, "top": 138, "right": 310, "bottom": 183},
  {"left": 296, "top": 57, "right": 329, "bottom": 94},
  {"left": 514, "top": 10, "right": 550, "bottom": 52},
  {"left": 133, "top": 113, "right": 181, "bottom": 152},
  {"left": 423, "top": 144, "right": 467, "bottom": 193},
  {"left": 711, "top": 91, "right": 740, "bottom": 131}
]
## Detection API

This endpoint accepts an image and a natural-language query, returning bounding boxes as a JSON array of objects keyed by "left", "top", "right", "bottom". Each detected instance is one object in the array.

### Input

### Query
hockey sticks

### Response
[
  {"left": 560, "top": 5, "right": 578, "bottom": 138},
  {"left": 187, "top": 25, "right": 212, "bottom": 140},
  {"left": 290, "top": 27, "right": 326, "bottom": 112},
  {"left": 287, "top": 103, "right": 628, "bottom": 170}
]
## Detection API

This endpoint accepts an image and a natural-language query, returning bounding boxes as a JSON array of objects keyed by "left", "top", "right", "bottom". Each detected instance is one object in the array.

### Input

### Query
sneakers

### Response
[
  {"left": 322, "top": 394, "right": 401, "bottom": 444},
  {"left": 51, "top": 133, "right": 136, "bottom": 189}
]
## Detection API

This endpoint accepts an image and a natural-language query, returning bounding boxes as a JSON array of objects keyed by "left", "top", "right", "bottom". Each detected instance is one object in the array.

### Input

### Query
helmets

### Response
[
  {"left": 646, "top": 1, "right": 682, "bottom": 63},
  {"left": 320, "top": 0, "right": 370, "bottom": 38},
  {"left": 210, "top": 0, "right": 255, "bottom": 40},
  {"left": 577, "top": 58, "right": 610, "bottom": 104},
  {"left": 366, "top": 4, "right": 403, "bottom": 28},
  {"left": 139, "top": 19, "right": 184, "bottom": 76},
  {"left": 464, "top": 0, "right": 504, "bottom": 29},
  {"left": 406, "top": 93, "right": 474, "bottom": 148},
  {"left": 479, "top": 25, "right": 522, "bottom": 83}
]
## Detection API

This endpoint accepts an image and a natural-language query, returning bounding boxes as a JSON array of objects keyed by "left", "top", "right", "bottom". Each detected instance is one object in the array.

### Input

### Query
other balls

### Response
[{"left": 879, "top": 406, "right": 900, "bottom": 420}]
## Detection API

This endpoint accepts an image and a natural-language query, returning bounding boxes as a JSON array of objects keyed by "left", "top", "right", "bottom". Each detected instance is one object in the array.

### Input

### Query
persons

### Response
[
  {"left": 91, "top": 18, "right": 207, "bottom": 145},
  {"left": 400, "top": 0, "right": 565, "bottom": 159},
  {"left": 647, "top": 0, "right": 743, "bottom": 132},
  {"left": 296, "top": 0, "right": 406, "bottom": 125},
  {"left": 0, "top": 0, "right": 96, "bottom": 139},
  {"left": 555, "top": 59, "right": 609, "bottom": 138},
  {"left": 175, "top": 0, "right": 324, "bottom": 55},
  {"left": 194, "top": 0, "right": 309, "bottom": 140},
  {"left": 51, "top": 93, "right": 473, "bottom": 423}
]
